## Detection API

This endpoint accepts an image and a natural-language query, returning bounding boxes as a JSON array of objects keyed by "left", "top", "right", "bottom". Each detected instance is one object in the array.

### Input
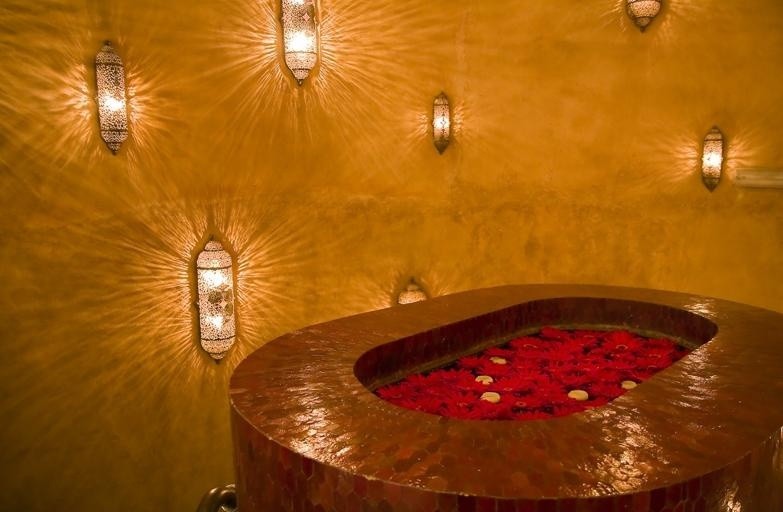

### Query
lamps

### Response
[
  {"left": 625, "top": 0, "right": 662, "bottom": 33},
  {"left": 281, "top": 0, "right": 317, "bottom": 85},
  {"left": 196, "top": 234, "right": 236, "bottom": 362},
  {"left": 432, "top": 91, "right": 451, "bottom": 154},
  {"left": 398, "top": 276, "right": 427, "bottom": 304},
  {"left": 701, "top": 126, "right": 723, "bottom": 192},
  {"left": 96, "top": 39, "right": 128, "bottom": 154}
]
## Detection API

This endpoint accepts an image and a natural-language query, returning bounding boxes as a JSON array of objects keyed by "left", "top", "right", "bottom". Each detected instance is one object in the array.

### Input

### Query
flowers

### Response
[{"left": 375, "top": 326, "right": 691, "bottom": 422}]
